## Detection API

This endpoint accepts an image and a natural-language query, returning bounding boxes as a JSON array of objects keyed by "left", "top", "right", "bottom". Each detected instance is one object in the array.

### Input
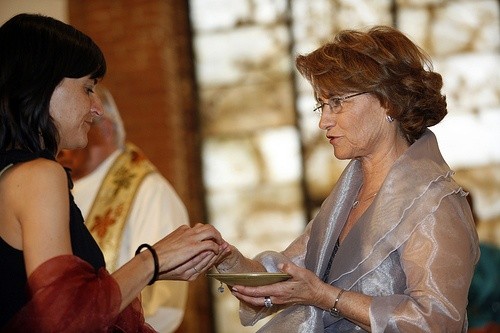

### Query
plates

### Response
[{"left": 204, "top": 274, "right": 292, "bottom": 287}]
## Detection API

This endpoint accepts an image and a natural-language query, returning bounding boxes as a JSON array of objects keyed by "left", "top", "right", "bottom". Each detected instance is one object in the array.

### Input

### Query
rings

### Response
[
  {"left": 264, "top": 297, "right": 273, "bottom": 308},
  {"left": 193, "top": 266, "right": 199, "bottom": 274}
]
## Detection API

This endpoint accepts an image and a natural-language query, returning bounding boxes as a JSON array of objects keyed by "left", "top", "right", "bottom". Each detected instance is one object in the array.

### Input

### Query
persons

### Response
[
  {"left": 0, "top": 13, "right": 231, "bottom": 333},
  {"left": 201, "top": 25, "right": 481, "bottom": 333},
  {"left": 56, "top": 83, "right": 191, "bottom": 333}
]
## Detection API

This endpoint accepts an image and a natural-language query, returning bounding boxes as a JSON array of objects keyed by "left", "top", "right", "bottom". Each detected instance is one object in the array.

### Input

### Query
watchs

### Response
[{"left": 329, "top": 289, "right": 345, "bottom": 319}]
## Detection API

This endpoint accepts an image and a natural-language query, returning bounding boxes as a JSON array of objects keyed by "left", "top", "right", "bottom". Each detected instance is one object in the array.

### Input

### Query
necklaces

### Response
[{"left": 352, "top": 191, "right": 378, "bottom": 208}]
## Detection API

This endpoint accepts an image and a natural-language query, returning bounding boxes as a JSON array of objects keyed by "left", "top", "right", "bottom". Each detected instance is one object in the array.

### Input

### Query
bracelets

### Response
[
  {"left": 135, "top": 243, "right": 159, "bottom": 286},
  {"left": 216, "top": 251, "right": 240, "bottom": 293}
]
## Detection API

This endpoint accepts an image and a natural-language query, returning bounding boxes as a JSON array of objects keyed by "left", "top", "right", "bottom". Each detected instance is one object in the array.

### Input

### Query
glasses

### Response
[{"left": 313, "top": 90, "right": 372, "bottom": 117}]
